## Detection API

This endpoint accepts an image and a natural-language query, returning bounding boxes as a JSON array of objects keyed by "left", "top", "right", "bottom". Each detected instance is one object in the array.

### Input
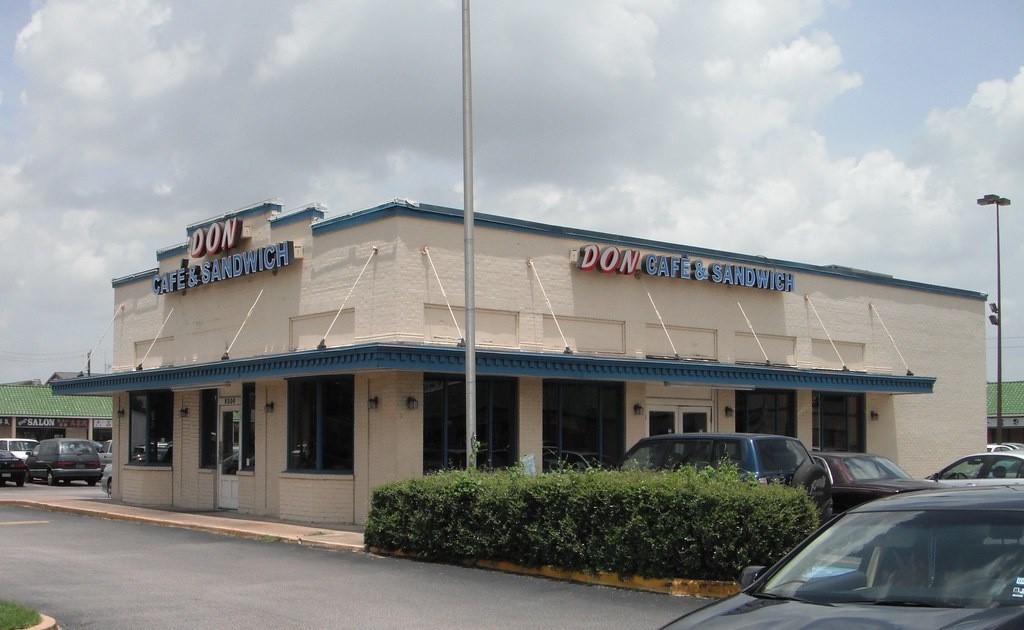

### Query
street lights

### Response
[{"left": 977, "top": 193, "right": 1013, "bottom": 446}]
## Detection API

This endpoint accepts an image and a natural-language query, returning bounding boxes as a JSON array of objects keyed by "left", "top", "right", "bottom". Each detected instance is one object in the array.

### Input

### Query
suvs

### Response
[{"left": 615, "top": 434, "right": 832, "bottom": 525}]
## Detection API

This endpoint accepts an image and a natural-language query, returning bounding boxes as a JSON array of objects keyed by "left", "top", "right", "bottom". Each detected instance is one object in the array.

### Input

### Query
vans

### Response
[
  {"left": 23, "top": 438, "right": 103, "bottom": 486},
  {"left": 0, "top": 437, "right": 41, "bottom": 464}
]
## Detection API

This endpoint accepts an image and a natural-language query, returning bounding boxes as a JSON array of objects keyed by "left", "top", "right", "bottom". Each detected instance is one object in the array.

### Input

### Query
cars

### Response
[
  {"left": 0, "top": 449, "right": 26, "bottom": 487},
  {"left": 100, "top": 463, "right": 114, "bottom": 498},
  {"left": 658, "top": 487, "right": 1024, "bottom": 630},
  {"left": 96, "top": 438, "right": 113, "bottom": 468},
  {"left": 88, "top": 441, "right": 103, "bottom": 449},
  {"left": 919, "top": 451, "right": 1024, "bottom": 487},
  {"left": 419, "top": 439, "right": 617, "bottom": 477},
  {"left": 809, "top": 450, "right": 953, "bottom": 516},
  {"left": 129, "top": 437, "right": 313, "bottom": 470},
  {"left": 984, "top": 440, "right": 1024, "bottom": 453}
]
